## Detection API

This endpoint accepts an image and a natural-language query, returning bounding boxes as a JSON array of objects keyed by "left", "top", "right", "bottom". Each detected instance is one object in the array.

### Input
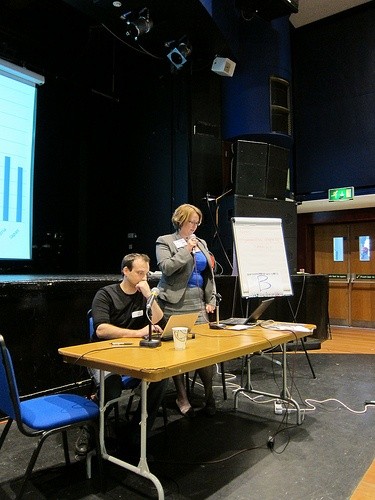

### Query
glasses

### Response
[
  {"left": 130, "top": 269, "right": 149, "bottom": 277},
  {"left": 187, "top": 220, "right": 200, "bottom": 226}
]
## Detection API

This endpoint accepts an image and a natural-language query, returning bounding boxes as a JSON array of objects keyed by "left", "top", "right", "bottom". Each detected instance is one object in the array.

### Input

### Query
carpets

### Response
[{"left": 0, "top": 350, "right": 375, "bottom": 500}]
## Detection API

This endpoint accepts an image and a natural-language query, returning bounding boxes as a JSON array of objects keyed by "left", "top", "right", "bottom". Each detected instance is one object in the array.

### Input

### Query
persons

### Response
[
  {"left": 74, "top": 253, "right": 169, "bottom": 456},
  {"left": 156, "top": 203, "right": 216, "bottom": 418}
]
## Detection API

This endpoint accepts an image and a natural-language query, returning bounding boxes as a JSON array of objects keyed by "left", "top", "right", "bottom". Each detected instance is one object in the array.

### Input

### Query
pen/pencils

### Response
[{"left": 111, "top": 343, "right": 133, "bottom": 345}]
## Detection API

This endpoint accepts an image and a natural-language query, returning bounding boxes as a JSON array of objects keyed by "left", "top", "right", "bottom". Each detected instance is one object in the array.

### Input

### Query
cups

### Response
[
  {"left": 171, "top": 326, "right": 189, "bottom": 351},
  {"left": 300, "top": 268, "right": 305, "bottom": 273}
]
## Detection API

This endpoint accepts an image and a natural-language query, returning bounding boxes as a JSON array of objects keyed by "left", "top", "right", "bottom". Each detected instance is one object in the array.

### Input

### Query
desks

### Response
[{"left": 56, "top": 319, "right": 316, "bottom": 500}]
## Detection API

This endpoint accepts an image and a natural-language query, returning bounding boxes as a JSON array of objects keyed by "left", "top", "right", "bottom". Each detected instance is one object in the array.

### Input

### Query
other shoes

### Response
[
  {"left": 73, "top": 426, "right": 93, "bottom": 455},
  {"left": 133, "top": 429, "right": 140, "bottom": 445},
  {"left": 206, "top": 401, "right": 217, "bottom": 416},
  {"left": 176, "top": 399, "right": 192, "bottom": 415}
]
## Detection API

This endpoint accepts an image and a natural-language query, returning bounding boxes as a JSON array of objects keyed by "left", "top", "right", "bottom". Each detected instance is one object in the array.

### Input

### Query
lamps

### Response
[
  {"left": 212, "top": 57, "right": 236, "bottom": 77},
  {"left": 166, "top": 42, "right": 190, "bottom": 69},
  {"left": 121, "top": 6, "right": 153, "bottom": 40}
]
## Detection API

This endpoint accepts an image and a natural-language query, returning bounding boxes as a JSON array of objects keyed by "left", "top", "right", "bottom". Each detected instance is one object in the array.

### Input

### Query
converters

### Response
[{"left": 267, "top": 436, "right": 274, "bottom": 448}]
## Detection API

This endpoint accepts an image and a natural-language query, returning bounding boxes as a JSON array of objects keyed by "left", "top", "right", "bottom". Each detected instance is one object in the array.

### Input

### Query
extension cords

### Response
[{"left": 274, "top": 401, "right": 282, "bottom": 414}]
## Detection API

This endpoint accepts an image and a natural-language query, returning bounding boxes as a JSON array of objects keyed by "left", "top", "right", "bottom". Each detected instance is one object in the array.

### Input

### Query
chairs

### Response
[{"left": 0, "top": 334, "right": 98, "bottom": 500}]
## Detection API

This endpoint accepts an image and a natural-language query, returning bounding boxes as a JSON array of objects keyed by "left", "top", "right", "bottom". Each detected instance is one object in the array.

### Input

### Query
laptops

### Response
[
  {"left": 213, "top": 297, "right": 275, "bottom": 326},
  {"left": 143, "top": 312, "right": 199, "bottom": 341}
]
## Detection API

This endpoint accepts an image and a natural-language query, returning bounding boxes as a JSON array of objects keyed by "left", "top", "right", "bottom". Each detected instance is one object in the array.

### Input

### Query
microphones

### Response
[{"left": 146, "top": 286, "right": 160, "bottom": 309}]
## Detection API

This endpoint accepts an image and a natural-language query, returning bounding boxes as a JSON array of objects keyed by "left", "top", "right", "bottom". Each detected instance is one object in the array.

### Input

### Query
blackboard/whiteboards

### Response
[{"left": 230, "top": 217, "right": 294, "bottom": 298}]
